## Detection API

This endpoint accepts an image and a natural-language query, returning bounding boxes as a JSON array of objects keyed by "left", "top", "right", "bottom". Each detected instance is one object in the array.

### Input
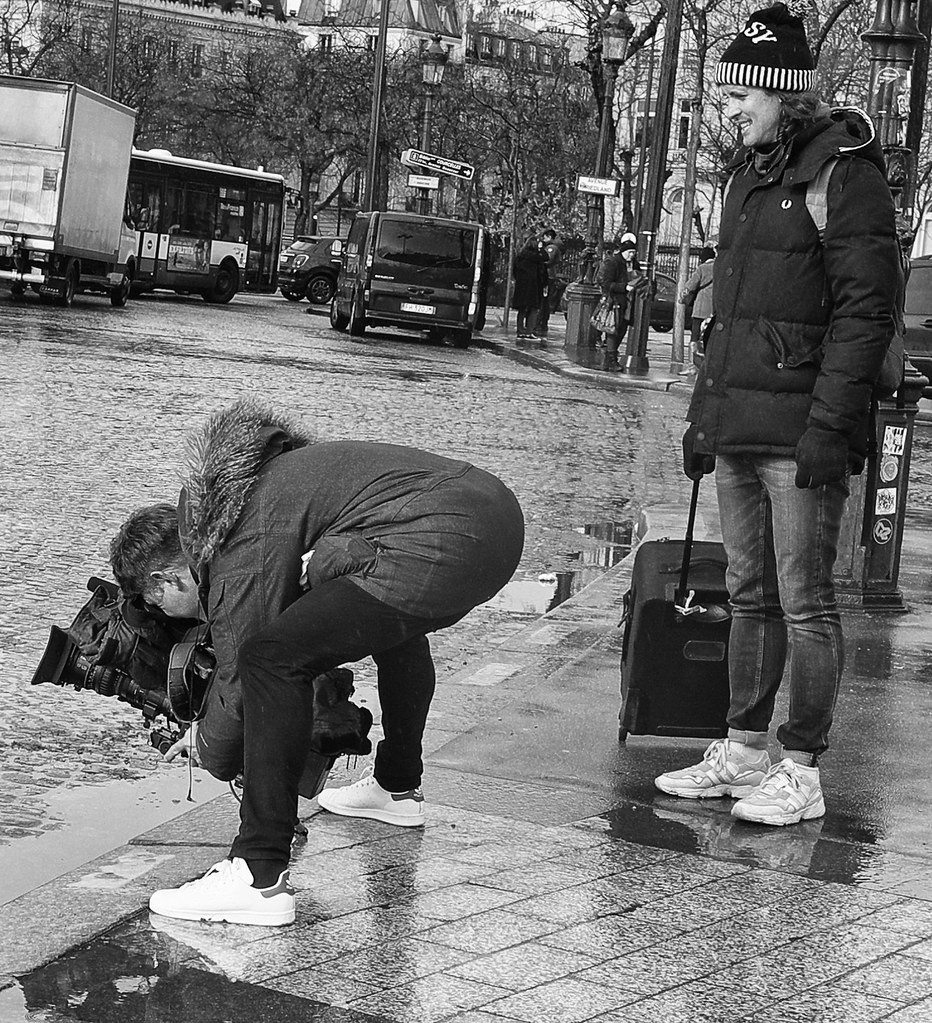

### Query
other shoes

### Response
[
  {"left": 524, "top": 333, "right": 540, "bottom": 341},
  {"left": 535, "top": 325, "right": 548, "bottom": 332},
  {"left": 517, "top": 333, "right": 525, "bottom": 339}
]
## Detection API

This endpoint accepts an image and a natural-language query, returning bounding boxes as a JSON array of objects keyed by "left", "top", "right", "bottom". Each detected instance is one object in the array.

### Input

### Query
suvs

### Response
[{"left": 278, "top": 236, "right": 351, "bottom": 304}]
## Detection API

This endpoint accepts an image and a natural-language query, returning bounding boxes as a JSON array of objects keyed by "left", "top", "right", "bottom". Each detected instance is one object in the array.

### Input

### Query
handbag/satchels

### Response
[
  {"left": 682, "top": 289, "right": 699, "bottom": 306},
  {"left": 589, "top": 283, "right": 619, "bottom": 335}
]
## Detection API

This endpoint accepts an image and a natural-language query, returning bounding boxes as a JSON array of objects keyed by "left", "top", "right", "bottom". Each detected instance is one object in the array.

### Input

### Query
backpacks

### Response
[{"left": 724, "top": 155, "right": 910, "bottom": 404}]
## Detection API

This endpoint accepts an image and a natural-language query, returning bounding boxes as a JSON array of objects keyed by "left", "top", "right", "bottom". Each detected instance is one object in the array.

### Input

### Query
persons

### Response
[
  {"left": 111, "top": 398, "right": 526, "bottom": 926},
  {"left": 598, "top": 241, "right": 642, "bottom": 371},
  {"left": 677, "top": 247, "right": 716, "bottom": 374},
  {"left": 193, "top": 240, "right": 210, "bottom": 270},
  {"left": 512, "top": 230, "right": 560, "bottom": 341},
  {"left": 653, "top": 3, "right": 906, "bottom": 825},
  {"left": 655, "top": 797, "right": 823, "bottom": 873}
]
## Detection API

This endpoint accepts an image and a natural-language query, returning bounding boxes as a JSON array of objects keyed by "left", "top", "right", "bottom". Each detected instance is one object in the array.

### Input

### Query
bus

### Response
[{"left": 127, "top": 146, "right": 306, "bottom": 305}]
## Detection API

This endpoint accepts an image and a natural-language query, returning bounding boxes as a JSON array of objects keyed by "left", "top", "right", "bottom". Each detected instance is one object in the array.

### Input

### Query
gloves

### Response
[
  {"left": 682, "top": 424, "right": 715, "bottom": 481},
  {"left": 794, "top": 425, "right": 845, "bottom": 489}
]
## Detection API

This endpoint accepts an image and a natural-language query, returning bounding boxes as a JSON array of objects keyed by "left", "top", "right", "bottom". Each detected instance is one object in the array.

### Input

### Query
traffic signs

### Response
[{"left": 407, "top": 148, "right": 474, "bottom": 181}]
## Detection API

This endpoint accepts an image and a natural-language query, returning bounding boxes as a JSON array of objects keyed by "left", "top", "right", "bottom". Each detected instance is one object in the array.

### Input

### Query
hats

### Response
[
  {"left": 620, "top": 240, "right": 636, "bottom": 253},
  {"left": 714, "top": 3, "right": 816, "bottom": 93}
]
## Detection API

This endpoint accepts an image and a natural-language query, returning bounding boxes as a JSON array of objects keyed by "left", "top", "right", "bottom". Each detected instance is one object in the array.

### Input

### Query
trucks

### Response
[{"left": 0, "top": 74, "right": 153, "bottom": 307}]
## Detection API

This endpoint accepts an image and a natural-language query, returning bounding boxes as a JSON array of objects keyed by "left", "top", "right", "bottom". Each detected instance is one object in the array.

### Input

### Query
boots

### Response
[
  {"left": 603, "top": 351, "right": 623, "bottom": 370},
  {"left": 677, "top": 341, "right": 697, "bottom": 376}
]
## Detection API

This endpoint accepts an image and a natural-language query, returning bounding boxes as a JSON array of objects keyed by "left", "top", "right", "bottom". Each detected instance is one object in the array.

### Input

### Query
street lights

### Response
[
  {"left": 576, "top": 9, "right": 636, "bottom": 284},
  {"left": 420, "top": 31, "right": 447, "bottom": 215},
  {"left": 397, "top": 235, "right": 414, "bottom": 255}
]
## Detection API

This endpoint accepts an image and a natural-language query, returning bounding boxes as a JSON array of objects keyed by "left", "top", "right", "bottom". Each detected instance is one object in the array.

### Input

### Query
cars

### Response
[
  {"left": 562, "top": 269, "right": 694, "bottom": 333},
  {"left": 902, "top": 255, "right": 932, "bottom": 393}
]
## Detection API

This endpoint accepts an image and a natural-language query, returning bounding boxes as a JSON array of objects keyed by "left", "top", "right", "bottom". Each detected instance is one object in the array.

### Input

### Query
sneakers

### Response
[
  {"left": 149, "top": 858, "right": 296, "bottom": 927},
  {"left": 317, "top": 766, "right": 424, "bottom": 826},
  {"left": 730, "top": 758, "right": 826, "bottom": 825},
  {"left": 655, "top": 738, "right": 772, "bottom": 799}
]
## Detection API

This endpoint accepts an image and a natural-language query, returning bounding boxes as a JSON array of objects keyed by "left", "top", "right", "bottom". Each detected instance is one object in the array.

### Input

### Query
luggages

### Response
[{"left": 618, "top": 476, "right": 732, "bottom": 742}]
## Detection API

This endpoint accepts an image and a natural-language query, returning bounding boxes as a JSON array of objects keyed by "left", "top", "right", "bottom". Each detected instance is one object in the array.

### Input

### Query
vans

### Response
[{"left": 327, "top": 214, "right": 495, "bottom": 347}]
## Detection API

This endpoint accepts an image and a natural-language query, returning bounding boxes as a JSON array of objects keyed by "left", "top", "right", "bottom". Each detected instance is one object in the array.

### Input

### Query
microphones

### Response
[{"left": 86, "top": 577, "right": 121, "bottom": 601}]
